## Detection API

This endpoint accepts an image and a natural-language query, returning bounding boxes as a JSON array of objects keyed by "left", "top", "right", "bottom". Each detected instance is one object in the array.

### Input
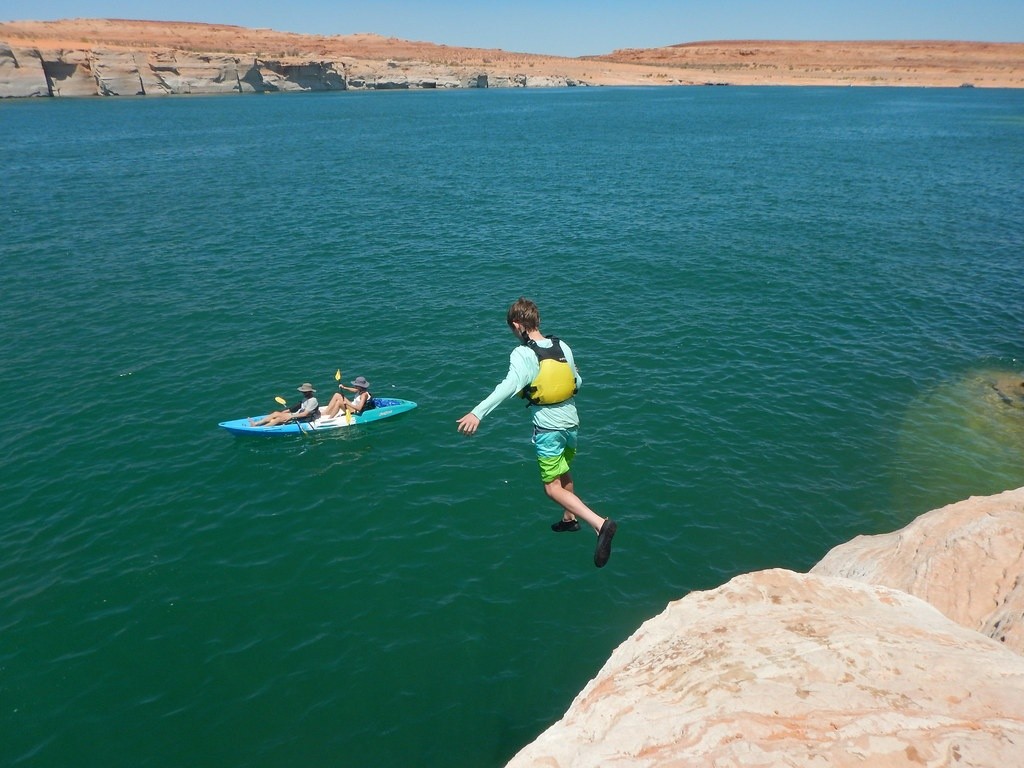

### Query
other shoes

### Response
[
  {"left": 551, "top": 518, "right": 580, "bottom": 532},
  {"left": 593, "top": 517, "right": 617, "bottom": 568}
]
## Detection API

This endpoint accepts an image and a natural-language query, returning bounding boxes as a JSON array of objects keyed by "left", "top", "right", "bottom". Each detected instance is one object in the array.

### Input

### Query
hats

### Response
[
  {"left": 296, "top": 382, "right": 317, "bottom": 392},
  {"left": 350, "top": 376, "right": 369, "bottom": 388}
]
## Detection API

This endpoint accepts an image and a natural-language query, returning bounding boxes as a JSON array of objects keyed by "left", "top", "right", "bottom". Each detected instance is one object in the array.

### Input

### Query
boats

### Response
[{"left": 218, "top": 398, "right": 416, "bottom": 435}]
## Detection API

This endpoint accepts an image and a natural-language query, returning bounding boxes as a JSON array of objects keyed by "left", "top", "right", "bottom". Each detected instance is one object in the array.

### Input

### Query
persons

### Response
[
  {"left": 320, "top": 376, "right": 376, "bottom": 419},
  {"left": 456, "top": 296, "right": 617, "bottom": 568},
  {"left": 248, "top": 382, "right": 319, "bottom": 428}
]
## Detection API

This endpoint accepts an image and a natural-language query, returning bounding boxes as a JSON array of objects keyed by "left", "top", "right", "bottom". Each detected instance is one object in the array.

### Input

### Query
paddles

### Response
[
  {"left": 274, "top": 397, "right": 307, "bottom": 434},
  {"left": 335, "top": 368, "right": 351, "bottom": 424}
]
